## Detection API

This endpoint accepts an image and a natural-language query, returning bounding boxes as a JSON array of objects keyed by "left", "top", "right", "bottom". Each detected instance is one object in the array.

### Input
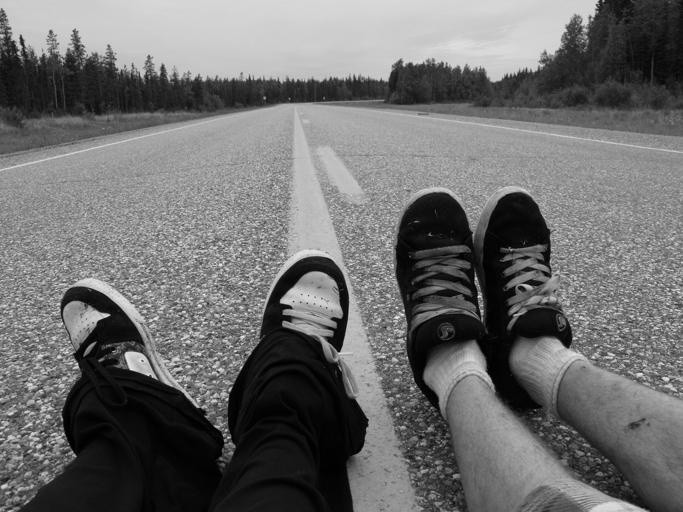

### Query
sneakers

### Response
[
  {"left": 396, "top": 184, "right": 492, "bottom": 409},
  {"left": 60, "top": 278, "right": 199, "bottom": 409},
  {"left": 258, "top": 247, "right": 349, "bottom": 353},
  {"left": 474, "top": 185, "right": 573, "bottom": 412}
]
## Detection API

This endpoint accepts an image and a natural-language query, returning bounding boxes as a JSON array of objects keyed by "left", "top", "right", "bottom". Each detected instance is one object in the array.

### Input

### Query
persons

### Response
[
  {"left": 18, "top": 247, "right": 370, "bottom": 511},
  {"left": 392, "top": 184, "right": 682, "bottom": 511}
]
